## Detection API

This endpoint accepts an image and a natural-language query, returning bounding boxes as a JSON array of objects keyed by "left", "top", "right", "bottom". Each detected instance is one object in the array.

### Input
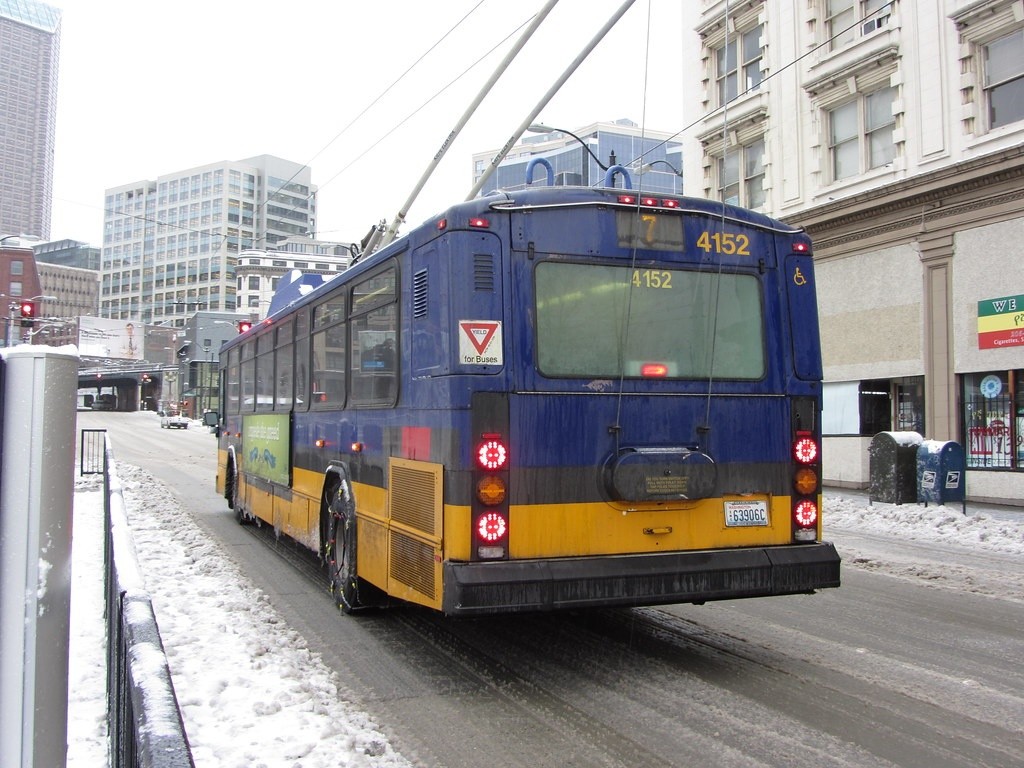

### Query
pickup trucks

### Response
[
  {"left": 160, "top": 410, "right": 189, "bottom": 430},
  {"left": 91, "top": 399, "right": 113, "bottom": 411}
]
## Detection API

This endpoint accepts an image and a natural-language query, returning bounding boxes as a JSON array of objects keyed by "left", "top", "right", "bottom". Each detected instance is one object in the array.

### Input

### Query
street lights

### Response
[
  {"left": 635, "top": 159, "right": 683, "bottom": 178},
  {"left": 527, "top": 124, "right": 617, "bottom": 187},
  {"left": 164, "top": 372, "right": 176, "bottom": 400},
  {"left": 164, "top": 346, "right": 186, "bottom": 410},
  {"left": 182, "top": 340, "right": 211, "bottom": 410}
]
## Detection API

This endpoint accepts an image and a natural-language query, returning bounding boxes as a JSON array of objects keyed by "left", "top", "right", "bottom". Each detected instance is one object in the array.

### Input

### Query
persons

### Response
[
  {"left": 375, "top": 338, "right": 395, "bottom": 399},
  {"left": 120, "top": 324, "right": 138, "bottom": 358}
]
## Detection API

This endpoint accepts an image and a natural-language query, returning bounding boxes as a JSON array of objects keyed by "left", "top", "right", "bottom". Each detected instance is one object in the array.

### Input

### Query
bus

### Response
[{"left": 201, "top": 157, "right": 842, "bottom": 619}]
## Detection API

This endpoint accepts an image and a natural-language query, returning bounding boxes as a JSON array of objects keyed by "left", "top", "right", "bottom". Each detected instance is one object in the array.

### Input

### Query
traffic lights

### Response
[
  {"left": 21, "top": 299, "right": 34, "bottom": 328},
  {"left": 238, "top": 321, "right": 252, "bottom": 335}
]
